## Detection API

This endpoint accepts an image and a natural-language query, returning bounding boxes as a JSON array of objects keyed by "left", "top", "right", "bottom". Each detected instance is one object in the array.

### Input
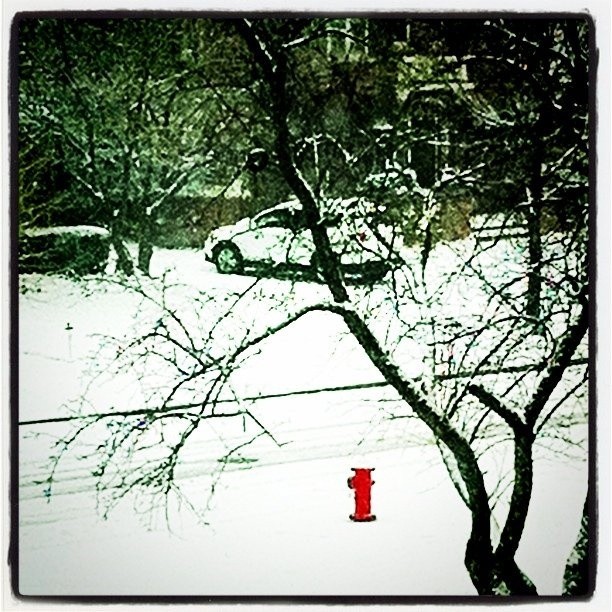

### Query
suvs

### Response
[{"left": 205, "top": 200, "right": 404, "bottom": 285}]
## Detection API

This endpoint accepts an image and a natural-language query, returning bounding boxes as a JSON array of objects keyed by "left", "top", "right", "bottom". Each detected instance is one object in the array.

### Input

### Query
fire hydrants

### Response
[{"left": 348, "top": 467, "right": 376, "bottom": 522}]
